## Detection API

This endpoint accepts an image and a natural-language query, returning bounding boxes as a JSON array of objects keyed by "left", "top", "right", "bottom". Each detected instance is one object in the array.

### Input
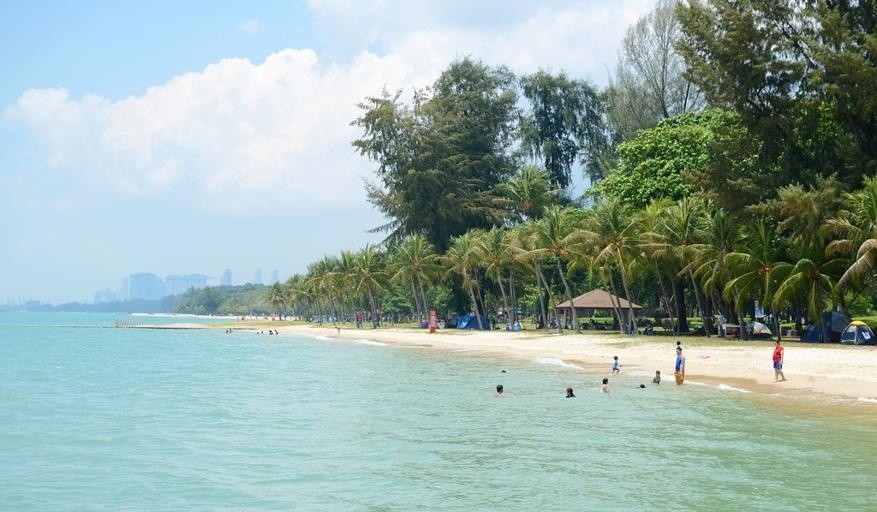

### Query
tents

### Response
[
  {"left": 741, "top": 320, "right": 772, "bottom": 339},
  {"left": 839, "top": 320, "right": 873, "bottom": 345},
  {"left": 442, "top": 313, "right": 490, "bottom": 332},
  {"left": 421, "top": 321, "right": 440, "bottom": 329},
  {"left": 800, "top": 309, "right": 850, "bottom": 344}
]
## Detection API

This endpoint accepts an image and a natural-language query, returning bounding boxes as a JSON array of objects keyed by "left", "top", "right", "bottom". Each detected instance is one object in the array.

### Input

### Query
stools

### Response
[{"left": 638, "top": 331, "right": 656, "bottom": 335}]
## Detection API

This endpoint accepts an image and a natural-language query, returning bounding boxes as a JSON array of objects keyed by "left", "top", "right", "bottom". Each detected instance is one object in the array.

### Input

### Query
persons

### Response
[
  {"left": 639, "top": 384, "right": 644, "bottom": 388},
  {"left": 771, "top": 339, "right": 785, "bottom": 382},
  {"left": 544, "top": 316, "right": 655, "bottom": 335},
  {"left": 673, "top": 347, "right": 685, "bottom": 385},
  {"left": 611, "top": 355, "right": 621, "bottom": 373},
  {"left": 255, "top": 329, "right": 278, "bottom": 337},
  {"left": 651, "top": 370, "right": 660, "bottom": 384},
  {"left": 355, "top": 313, "right": 363, "bottom": 329},
  {"left": 675, "top": 341, "right": 681, "bottom": 347},
  {"left": 495, "top": 384, "right": 504, "bottom": 398},
  {"left": 601, "top": 378, "right": 608, "bottom": 393},
  {"left": 565, "top": 387, "right": 576, "bottom": 398}
]
